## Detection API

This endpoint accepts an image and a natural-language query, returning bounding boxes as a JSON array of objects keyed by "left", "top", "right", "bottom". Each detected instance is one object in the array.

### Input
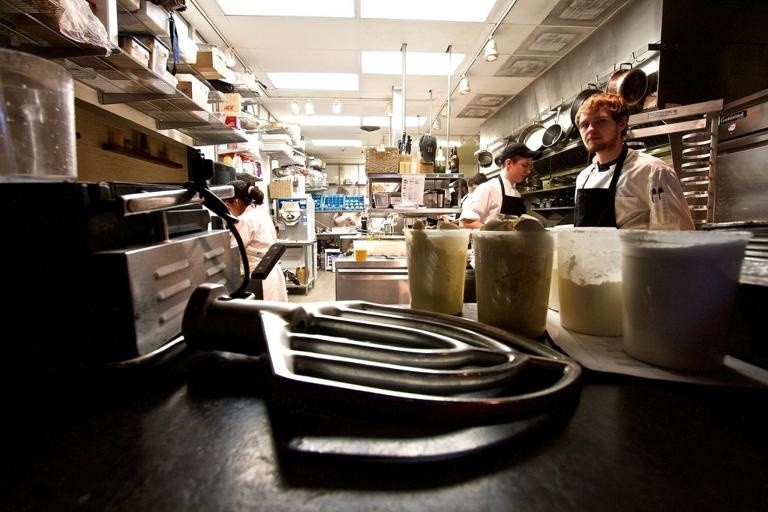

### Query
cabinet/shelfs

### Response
[
  {"left": 367, "top": 173, "right": 464, "bottom": 217},
  {"left": 473, "top": 39, "right": 660, "bottom": 211},
  {"left": 219, "top": 89, "right": 263, "bottom": 183},
  {"left": 260, "top": 127, "right": 297, "bottom": 165},
  {"left": 0, "top": 0, "right": 248, "bottom": 146},
  {"left": 278, "top": 240, "right": 317, "bottom": 296}
]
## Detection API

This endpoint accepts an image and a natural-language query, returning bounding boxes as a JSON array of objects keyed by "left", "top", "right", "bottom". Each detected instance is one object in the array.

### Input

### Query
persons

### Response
[
  {"left": 468, "top": 173, "right": 487, "bottom": 191},
  {"left": 572, "top": 93, "right": 694, "bottom": 230},
  {"left": 457, "top": 140, "right": 537, "bottom": 232},
  {"left": 448, "top": 180, "right": 471, "bottom": 208},
  {"left": 223, "top": 180, "right": 288, "bottom": 301}
]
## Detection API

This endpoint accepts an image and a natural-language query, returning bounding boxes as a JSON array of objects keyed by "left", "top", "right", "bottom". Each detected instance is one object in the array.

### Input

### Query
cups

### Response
[
  {"left": 618, "top": 228, "right": 752, "bottom": 373},
  {"left": 0, "top": 47, "right": 78, "bottom": 183},
  {"left": 403, "top": 226, "right": 472, "bottom": 317},
  {"left": 471, "top": 227, "right": 555, "bottom": 338},
  {"left": 356, "top": 250, "right": 367, "bottom": 262},
  {"left": 555, "top": 228, "right": 624, "bottom": 338}
]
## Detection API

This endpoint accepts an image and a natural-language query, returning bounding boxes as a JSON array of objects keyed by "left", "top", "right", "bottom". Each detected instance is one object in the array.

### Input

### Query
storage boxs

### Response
[
  {"left": 94, "top": 36, "right": 170, "bottom": 80},
  {"left": 167, "top": 52, "right": 226, "bottom": 80},
  {"left": 217, "top": 92, "right": 241, "bottom": 112},
  {"left": 117, "top": 1, "right": 171, "bottom": 36},
  {"left": 150, "top": 81, "right": 210, "bottom": 112}
]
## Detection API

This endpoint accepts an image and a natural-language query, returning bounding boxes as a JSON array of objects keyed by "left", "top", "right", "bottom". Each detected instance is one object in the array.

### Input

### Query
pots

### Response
[{"left": 476, "top": 62, "right": 649, "bottom": 168}]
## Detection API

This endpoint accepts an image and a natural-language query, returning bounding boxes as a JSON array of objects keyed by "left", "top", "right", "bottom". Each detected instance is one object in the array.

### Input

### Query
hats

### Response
[{"left": 500, "top": 143, "right": 544, "bottom": 164}]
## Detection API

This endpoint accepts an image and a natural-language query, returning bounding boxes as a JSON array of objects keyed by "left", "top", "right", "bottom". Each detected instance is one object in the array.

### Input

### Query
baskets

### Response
[
  {"left": 268, "top": 181, "right": 293, "bottom": 199},
  {"left": 364, "top": 146, "right": 399, "bottom": 173}
]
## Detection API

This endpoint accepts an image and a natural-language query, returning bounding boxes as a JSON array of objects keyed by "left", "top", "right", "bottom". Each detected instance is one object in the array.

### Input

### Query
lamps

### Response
[
  {"left": 290, "top": 97, "right": 393, "bottom": 117},
  {"left": 433, "top": 35, "right": 500, "bottom": 131}
]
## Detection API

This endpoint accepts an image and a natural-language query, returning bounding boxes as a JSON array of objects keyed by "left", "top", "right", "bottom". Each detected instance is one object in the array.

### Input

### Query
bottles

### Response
[
  {"left": 361, "top": 210, "right": 367, "bottom": 235},
  {"left": 437, "top": 189, "right": 444, "bottom": 207},
  {"left": 223, "top": 154, "right": 262, "bottom": 177},
  {"left": 449, "top": 146, "right": 454, "bottom": 170},
  {"left": 436, "top": 147, "right": 446, "bottom": 173},
  {"left": 451, "top": 147, "right": 459, "bottom": 173},
  {"left": 379, "top": 143, "right": 385, "bottom": 151},
  {"left": 297, "top": 266, "right": 309, "bottom": 284}
]
